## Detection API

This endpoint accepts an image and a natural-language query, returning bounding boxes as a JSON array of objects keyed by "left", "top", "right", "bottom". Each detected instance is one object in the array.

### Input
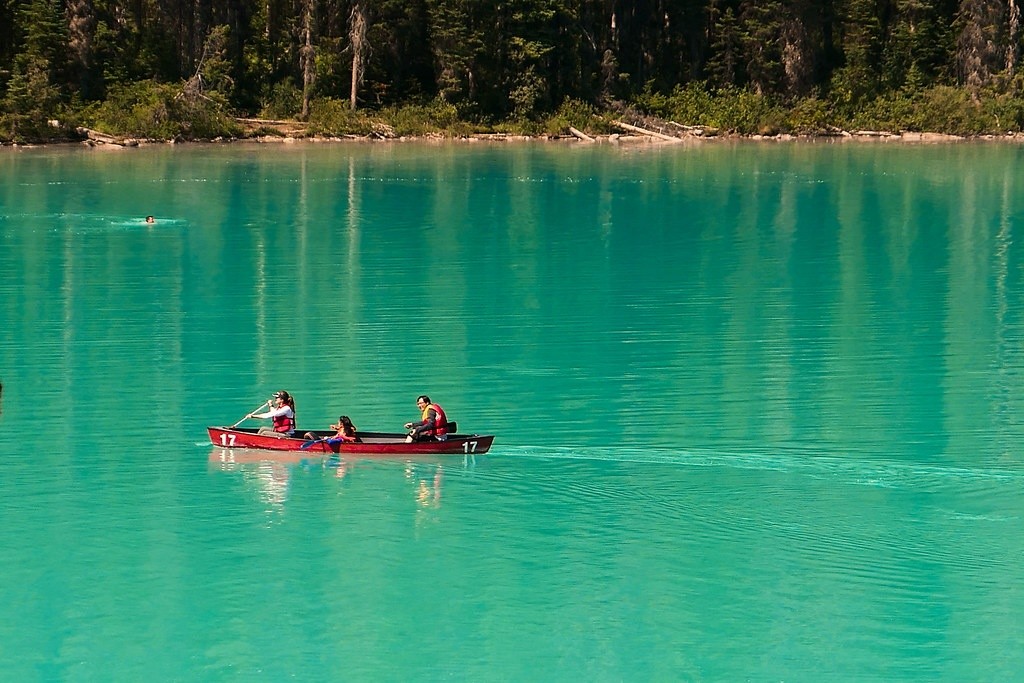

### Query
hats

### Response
[{"left": 271, "top": 391, "right": 288, "bottom": 401}]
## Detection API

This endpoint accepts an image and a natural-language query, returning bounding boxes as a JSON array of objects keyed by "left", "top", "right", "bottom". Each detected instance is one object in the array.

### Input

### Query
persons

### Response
[
  {"left": 145, "top": 216, "right": 154, "bottom": 223},
  {"left": 401, "top": 395, "right": 447, "bottom": 443},
  {"left": 246, "top": 390, "right": 295, "bottom": 439},
  {"left": 303, "top": 415, "right": 357, "bottom": 443}
]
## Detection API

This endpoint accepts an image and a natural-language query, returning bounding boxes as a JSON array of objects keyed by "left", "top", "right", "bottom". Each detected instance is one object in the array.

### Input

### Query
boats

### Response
[{"left": 204, "top": 425, "right": 495, "bottom": 454}]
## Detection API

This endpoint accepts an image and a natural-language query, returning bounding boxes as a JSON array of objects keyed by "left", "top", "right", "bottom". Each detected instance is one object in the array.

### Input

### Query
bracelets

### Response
[{"left": 271, "top": 404, "right": 274, "bottom": 407}]
